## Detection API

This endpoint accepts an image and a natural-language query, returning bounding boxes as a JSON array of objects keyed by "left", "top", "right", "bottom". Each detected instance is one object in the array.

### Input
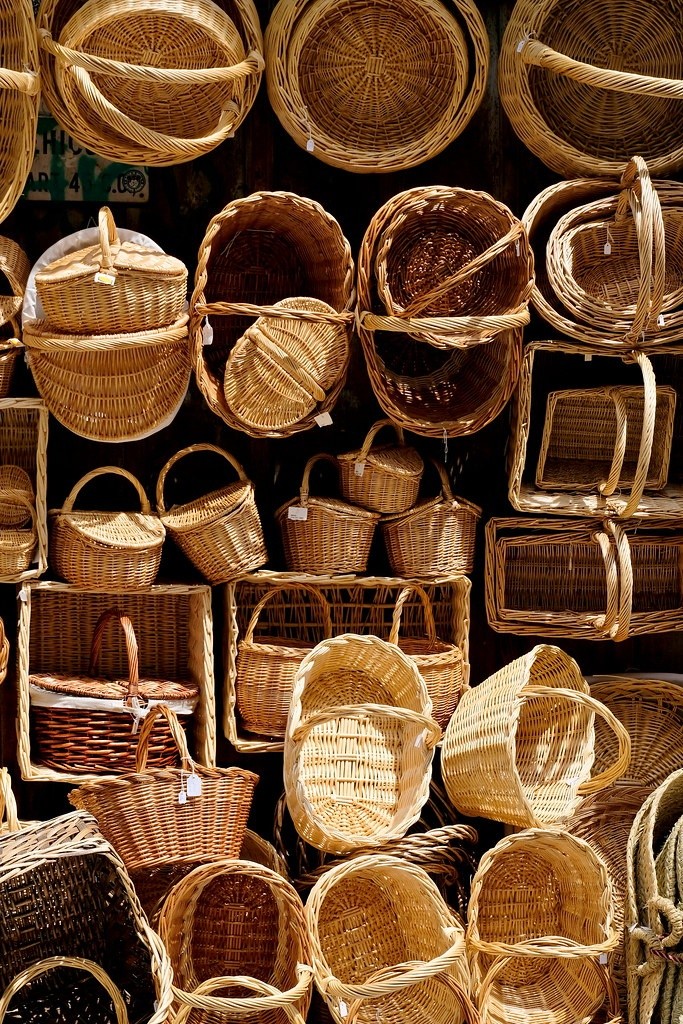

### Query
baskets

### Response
[{"left": 0, "top": 0, "right": 683, "bottom": 1023}]
